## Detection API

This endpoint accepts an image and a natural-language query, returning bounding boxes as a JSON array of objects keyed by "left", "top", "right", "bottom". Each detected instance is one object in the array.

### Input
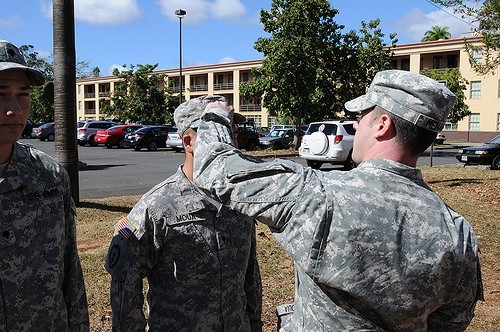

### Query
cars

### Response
[
  {"left": 166, "top": 132, "right": 184, "bottom": 150},
  {"left": 234, "top": 122, "right": 297, "bottom": 150},
  {"left": 436, "top": 134, "right": 446, "bottom": 145},
  {"left": 32, "top": 122, "right": 55, "bottom": 141},
  {"left": 22, "top": 120, "right": 43, "bottom": 137},
  {"left": 124, "top": 126, "right": 178, "bottom": 151},
  {"left": 456, "top": 135, "right": 500, "bottom": 170},
  {"left": 95, "top": 124, "right": 143, "bottom": 148}
]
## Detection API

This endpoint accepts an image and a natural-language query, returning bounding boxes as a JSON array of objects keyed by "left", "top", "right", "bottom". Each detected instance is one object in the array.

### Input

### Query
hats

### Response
[
  {"left": 173, "top": 95, "right": 246, "bottom": 138},
  {"left": 0, "top": 39, "right": 45, "bottom": 86},
  {"left": 344, "top": 70, "right": 458, "bottom": 134}
]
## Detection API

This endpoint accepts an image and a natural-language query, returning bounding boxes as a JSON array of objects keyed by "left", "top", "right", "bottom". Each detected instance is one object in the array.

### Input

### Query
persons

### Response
[
  {"left": 0, "top": 40, "right": 90, "bottom": 332},
  {"left": 104, "top": 95, "right": 262, "bottom": 332},
  {"left": 193, "top": 70, "right": 485, "bottom": 332}
]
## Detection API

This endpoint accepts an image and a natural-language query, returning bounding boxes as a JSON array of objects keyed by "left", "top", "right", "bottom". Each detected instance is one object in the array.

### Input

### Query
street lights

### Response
[{"left": 175, "top": 9, "right": 186, "bottom": 103}]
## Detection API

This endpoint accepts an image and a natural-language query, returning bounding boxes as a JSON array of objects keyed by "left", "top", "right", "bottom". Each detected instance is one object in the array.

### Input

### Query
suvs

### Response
[
  {"left": 76, "top": 120, "right": 127, "bottom": 146},
  {"left": 299, "top": 121, "right": 358, "bottom": 169}
]
